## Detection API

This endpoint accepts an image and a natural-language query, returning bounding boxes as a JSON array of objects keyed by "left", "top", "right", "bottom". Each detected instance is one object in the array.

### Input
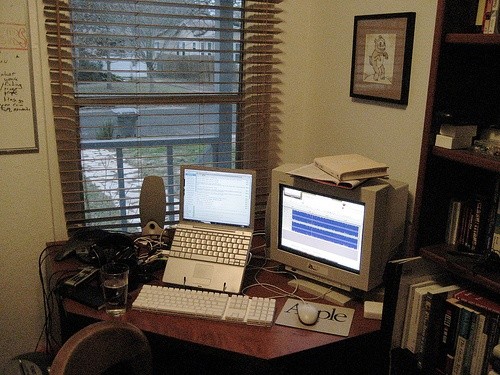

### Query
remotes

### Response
[{"left": 65, "top": 265, "right": 98, "bottom": 287}]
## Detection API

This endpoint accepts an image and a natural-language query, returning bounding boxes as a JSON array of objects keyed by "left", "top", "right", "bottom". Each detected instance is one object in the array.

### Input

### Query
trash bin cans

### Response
[{"left": 111, "top": 107, "right": 139, "bottom": 139}]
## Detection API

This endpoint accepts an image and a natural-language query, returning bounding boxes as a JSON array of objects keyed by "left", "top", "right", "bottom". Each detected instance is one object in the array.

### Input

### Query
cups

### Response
[{"left": 99, "top": 262, "right": 129, "bottom": 318}]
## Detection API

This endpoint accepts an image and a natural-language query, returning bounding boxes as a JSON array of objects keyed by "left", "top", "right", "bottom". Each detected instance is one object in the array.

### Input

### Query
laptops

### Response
[{"left": 162, "top": 164, "right": 257, "bottom": 293}]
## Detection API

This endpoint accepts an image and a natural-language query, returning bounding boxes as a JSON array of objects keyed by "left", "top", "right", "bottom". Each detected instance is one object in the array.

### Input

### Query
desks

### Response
[{"left": 42, "top": 239, "right": 386, "bottom": 366}]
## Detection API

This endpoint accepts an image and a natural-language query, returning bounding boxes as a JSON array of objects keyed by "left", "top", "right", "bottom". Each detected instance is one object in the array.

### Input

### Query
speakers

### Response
[{"left": 139, "top": 175, "right": 166, "bottom": 237}]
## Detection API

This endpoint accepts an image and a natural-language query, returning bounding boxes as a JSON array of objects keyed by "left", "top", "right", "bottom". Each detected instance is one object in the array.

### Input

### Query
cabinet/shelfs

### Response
[{"left": 385, "top": 0, "right": 500, "bottom": 375}]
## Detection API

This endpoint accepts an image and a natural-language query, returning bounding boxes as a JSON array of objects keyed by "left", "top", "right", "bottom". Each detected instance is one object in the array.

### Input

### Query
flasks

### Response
[{"left": 445, "top": 199, "right": 466, "bottom": 247}]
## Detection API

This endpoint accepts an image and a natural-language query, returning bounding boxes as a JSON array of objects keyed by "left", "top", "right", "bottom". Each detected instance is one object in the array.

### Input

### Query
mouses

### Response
[{"left": 296, "top": 302, "right": 319, "bottom": 326}]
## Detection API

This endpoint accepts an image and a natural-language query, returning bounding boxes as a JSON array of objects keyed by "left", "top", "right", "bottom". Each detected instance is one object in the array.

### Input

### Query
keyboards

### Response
[{"left": 131, "top": 284, "right": 277, "bottom": 327}]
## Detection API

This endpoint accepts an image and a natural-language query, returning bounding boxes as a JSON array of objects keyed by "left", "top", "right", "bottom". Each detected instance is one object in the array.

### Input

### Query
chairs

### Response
[{"left": 47, "top": 320, "right": 152, "bottom": 375}]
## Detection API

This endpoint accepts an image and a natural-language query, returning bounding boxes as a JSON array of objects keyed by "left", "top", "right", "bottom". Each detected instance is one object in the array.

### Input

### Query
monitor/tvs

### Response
[{"left": 269, "top": 162, "right": 409, "bottom": 306}]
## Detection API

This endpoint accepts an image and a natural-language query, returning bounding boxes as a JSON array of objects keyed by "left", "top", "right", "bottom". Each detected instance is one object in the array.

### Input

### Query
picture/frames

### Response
[{"left": 348, "top": 11, "right": 416, "bottom": 104}]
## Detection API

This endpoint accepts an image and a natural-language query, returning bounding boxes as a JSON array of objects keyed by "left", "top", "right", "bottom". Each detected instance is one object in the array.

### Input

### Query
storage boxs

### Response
[{"left": 435, "top": 122, "right": 478, "bottom": 149}]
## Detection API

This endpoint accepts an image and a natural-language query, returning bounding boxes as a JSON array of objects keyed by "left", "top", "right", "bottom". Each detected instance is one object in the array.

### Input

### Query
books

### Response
[
  {"left": 475, "top": 0, "right": 500, "bottom": 34},
  {"left": 315, "top": 153, "right": 390, "bottom": 180},
  {"left": 379, "top": 185, "right": 500, "bottom": 375}
]
